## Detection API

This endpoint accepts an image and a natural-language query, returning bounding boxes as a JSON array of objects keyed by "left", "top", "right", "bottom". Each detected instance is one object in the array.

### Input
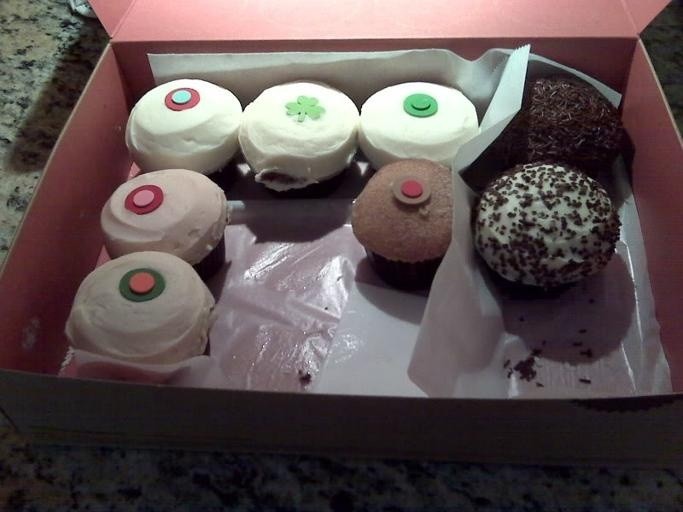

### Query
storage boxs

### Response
[{"left": 1, "top": 1, "right": 681, "bottom": 470}]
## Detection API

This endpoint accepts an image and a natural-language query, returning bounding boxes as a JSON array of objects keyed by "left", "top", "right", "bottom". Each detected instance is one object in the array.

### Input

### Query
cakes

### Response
[
  {"left": 491, "top": 78, "right": 623, "bottom": 169},
  {"left": 125, "top": 78, "right": 242, "bottom": 176},
  {"left": 99, "top": 167, "right": 228, "bottom": 280},
  {"left": 474, "top": 161, "right": 621, "bottom": 290},
  {"left": 238, "top": 82, "right": 360, "bottom": 191},
  {"left": 357, "top": 81, "right": 479, "bottom": 171},
  {"left": 352, "top": 159, "right": 453, "bottom": 286},
  {"left": 64, "top": 250, "right": 215, "bottom": 366}
]
